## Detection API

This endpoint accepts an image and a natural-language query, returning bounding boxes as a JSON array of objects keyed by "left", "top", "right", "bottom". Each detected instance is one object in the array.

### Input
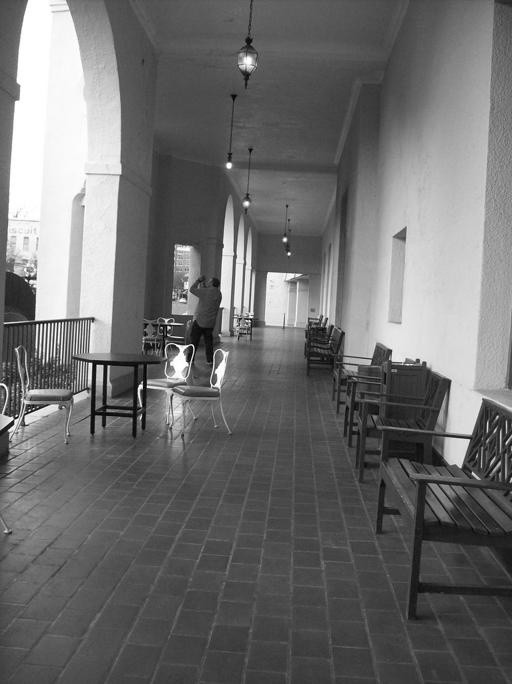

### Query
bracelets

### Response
[
  {"left": 197, "top": 277, "right": 201, "bottom": 282},
  {"left": 203, "top": 282, "right": 206, "bottom": 287}
]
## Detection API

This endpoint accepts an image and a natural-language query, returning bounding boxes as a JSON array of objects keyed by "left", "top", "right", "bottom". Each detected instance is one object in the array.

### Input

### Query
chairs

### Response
[
  {"left": 8, "top": 345, "right": 73, "bottom": 444},
  {"left": 170, "top": 348, "right": 232, "bottom": 437},
  {"left": 234, "top": 312, "right": 255, "bottom": 341},
  {"left": 137, "top": 343, "right": 197, "bottom": 424},
  {"left": 0, "top": 383, "right": 13, "bottom": 533},
  {"left": 142, "top": 317, "right": 192, "bottom": 355}
]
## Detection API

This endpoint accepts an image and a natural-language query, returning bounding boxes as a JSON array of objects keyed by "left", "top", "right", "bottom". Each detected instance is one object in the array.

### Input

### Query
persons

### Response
[{"left": 185, "top": 272, "right": 222, "bottom": 365}]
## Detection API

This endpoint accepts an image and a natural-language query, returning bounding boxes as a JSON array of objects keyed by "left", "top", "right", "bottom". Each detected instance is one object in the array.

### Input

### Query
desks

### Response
[{"left": 72, "top": 353, "right": 168, "bottom": 438}]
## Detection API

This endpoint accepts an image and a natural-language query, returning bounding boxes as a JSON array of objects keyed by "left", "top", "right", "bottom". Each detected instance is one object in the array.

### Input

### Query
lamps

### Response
[
  {"left": 242, "top": 148, "right": 253, "bottom": 213},
  {"left": 226, "top": 94, "right": 237, "bottom": 169},
  {"left": 237, "top": 0, "right": 259, "bottom": 90},
  {"left": 282, "top": 204, "right": 292, "bottom": 259}
]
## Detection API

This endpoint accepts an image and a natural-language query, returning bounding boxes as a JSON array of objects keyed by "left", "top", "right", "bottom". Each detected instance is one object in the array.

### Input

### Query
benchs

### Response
[
  {"left": 304, "top": 315, "right": 454, "bottom": 483},
  {"left": 377, "top": 397, "right": 512, "bottom": 621}
]
